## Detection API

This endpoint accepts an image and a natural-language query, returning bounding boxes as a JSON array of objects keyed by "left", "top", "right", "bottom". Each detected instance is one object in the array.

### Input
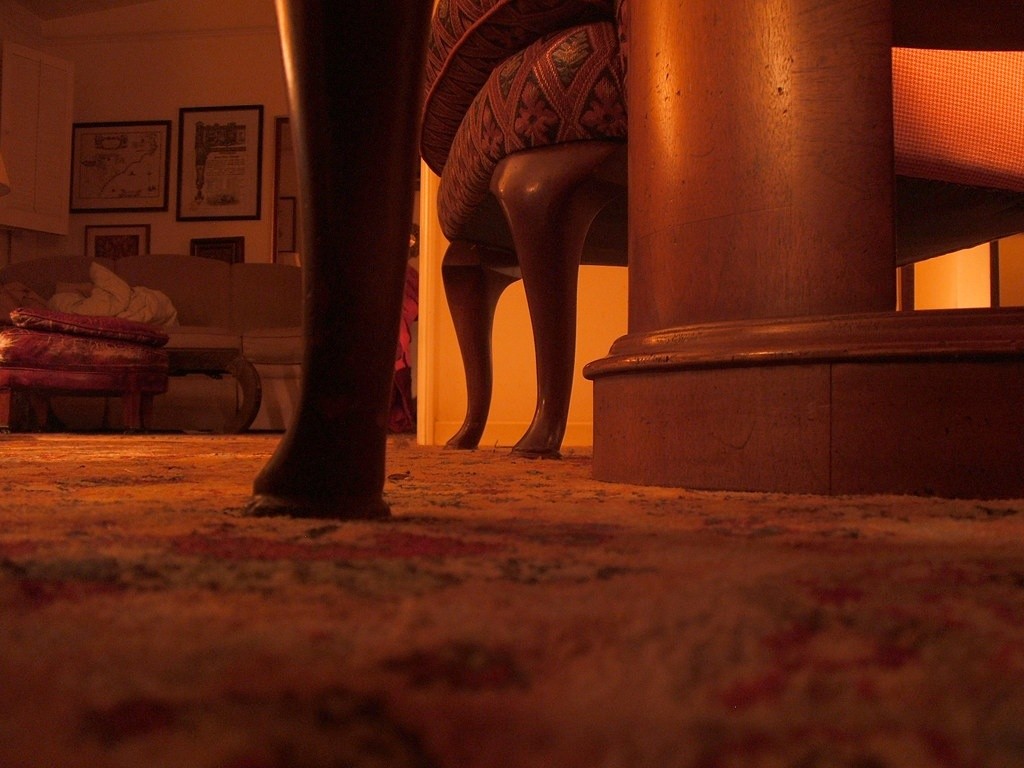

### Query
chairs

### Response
[{"left": 420, "top": 0, "right": 1024, "bottom": 460}]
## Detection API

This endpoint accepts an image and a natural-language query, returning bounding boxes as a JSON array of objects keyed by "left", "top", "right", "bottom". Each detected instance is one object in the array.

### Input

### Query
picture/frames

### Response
[
  {"left": 190, "top": 236, "right": 247, "bottom": 265},
  {"left": 175, "top": 104, "right": 264, "bottom": 222},
  {"left": 69, "top": 119, "right": 171, "bottom": 214},
  {"left": 275, "top": 197, "right": 296, "bottom": 254},
  {"left": 84, "top": 224, "right": 151, "bottom": 257}
]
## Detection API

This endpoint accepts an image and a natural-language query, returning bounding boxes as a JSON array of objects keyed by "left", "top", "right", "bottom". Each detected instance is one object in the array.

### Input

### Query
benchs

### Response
[{"left": 0, "top": 327, "right": 171, "bottom": 437}]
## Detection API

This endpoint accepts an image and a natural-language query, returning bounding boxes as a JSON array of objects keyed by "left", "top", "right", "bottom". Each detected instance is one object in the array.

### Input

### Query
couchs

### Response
[{"left": 0, "top": 253, "right": 306, "bottom": 436}]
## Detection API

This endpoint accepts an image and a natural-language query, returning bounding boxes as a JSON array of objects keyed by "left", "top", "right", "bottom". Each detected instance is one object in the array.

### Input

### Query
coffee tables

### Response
[{"left": 141, "top": 345, "right": 264, "bottom": 435}]
